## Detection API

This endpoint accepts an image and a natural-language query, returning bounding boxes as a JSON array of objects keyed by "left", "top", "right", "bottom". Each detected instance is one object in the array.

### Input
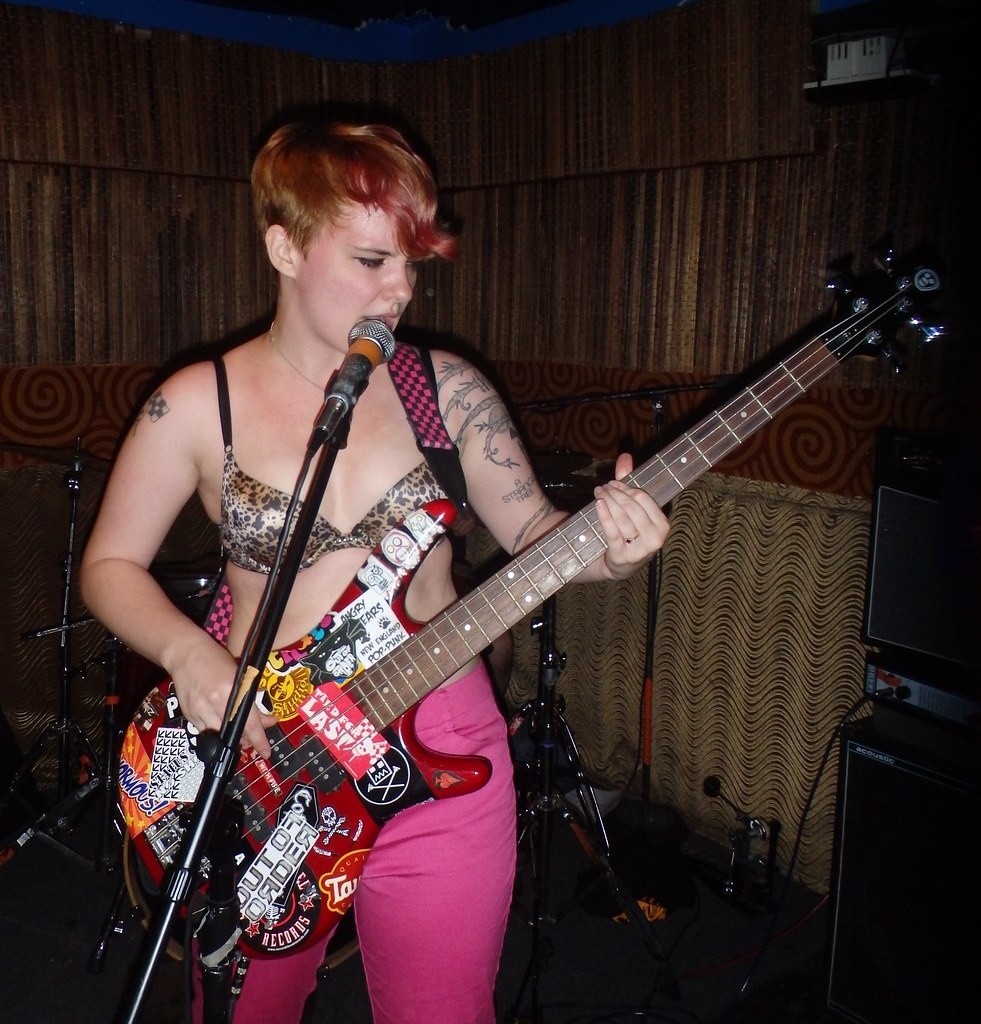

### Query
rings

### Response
[{"left": 622, "top": 535, "right": 639, "bottom": 545}]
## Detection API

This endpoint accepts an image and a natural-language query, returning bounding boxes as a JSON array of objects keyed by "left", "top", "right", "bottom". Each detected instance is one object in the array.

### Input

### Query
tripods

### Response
[
  {"left": 0, "top": 434, "right": 220, "bottom": 977},
  {"left": 500, "top": 591, "right": 682, "bottom": 1002}
]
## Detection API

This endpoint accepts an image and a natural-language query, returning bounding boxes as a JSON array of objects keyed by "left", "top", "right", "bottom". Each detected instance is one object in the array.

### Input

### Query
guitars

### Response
[{"left": 112, "top": 231, "right": 955, "bottom": 973}]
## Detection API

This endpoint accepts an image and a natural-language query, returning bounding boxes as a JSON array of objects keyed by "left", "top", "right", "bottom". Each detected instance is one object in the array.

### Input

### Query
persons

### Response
[{"left": 77, "top": 116, "right": 671, "bottom": 1023}]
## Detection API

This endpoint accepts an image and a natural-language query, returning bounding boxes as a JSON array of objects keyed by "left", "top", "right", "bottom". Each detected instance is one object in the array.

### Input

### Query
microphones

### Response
[{"left": 309, "top": 319, "right": 394, "bottom": 457}]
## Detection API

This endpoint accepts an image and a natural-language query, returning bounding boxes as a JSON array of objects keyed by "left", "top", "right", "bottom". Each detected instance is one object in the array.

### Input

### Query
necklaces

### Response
[{"left": 269, "top": 320, "right": 329, "bottom": 399}]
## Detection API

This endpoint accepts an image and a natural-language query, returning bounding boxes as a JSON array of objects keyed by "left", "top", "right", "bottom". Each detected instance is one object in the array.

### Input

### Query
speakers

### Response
[
  {"left": 859, "top": 480, "right": 981, "bottom": 677},
  {"left": 826, "top": 703, "right": 981, "bottom": 1024},
  {"left": 0, "top": 711, "right": 44, "bottom": 847}
]
